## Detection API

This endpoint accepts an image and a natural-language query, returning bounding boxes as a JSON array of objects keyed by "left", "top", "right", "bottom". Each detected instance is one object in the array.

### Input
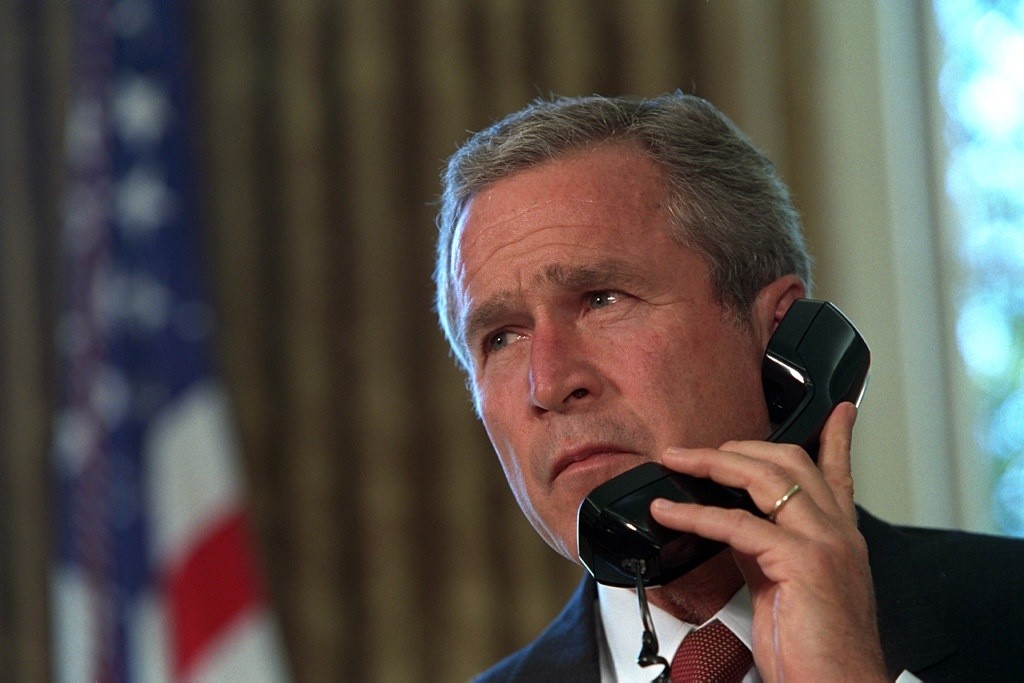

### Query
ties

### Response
[{"left": 669, "top": 621, "right": 755, "bottom": 683}]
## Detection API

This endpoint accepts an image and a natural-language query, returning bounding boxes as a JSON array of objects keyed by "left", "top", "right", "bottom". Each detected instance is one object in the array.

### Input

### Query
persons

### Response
[{"left": 434, "top": 89, "right": 1024, "bottom": 683}]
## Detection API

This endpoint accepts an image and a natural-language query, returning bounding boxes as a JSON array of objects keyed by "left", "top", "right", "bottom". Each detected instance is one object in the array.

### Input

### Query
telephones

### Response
[{"left": 574, "top": 297, "right": 875, "bottom": 592}]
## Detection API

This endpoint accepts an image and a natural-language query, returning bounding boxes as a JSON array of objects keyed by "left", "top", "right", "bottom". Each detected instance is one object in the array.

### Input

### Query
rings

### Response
[{"left": 770, "top": 484, "right": 803, "bottom": 521}]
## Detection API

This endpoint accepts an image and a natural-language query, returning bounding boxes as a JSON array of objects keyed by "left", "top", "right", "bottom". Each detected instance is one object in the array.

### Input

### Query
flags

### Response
[{"left": 44, "top": 0, "right": 286, "bottom": 683}]
